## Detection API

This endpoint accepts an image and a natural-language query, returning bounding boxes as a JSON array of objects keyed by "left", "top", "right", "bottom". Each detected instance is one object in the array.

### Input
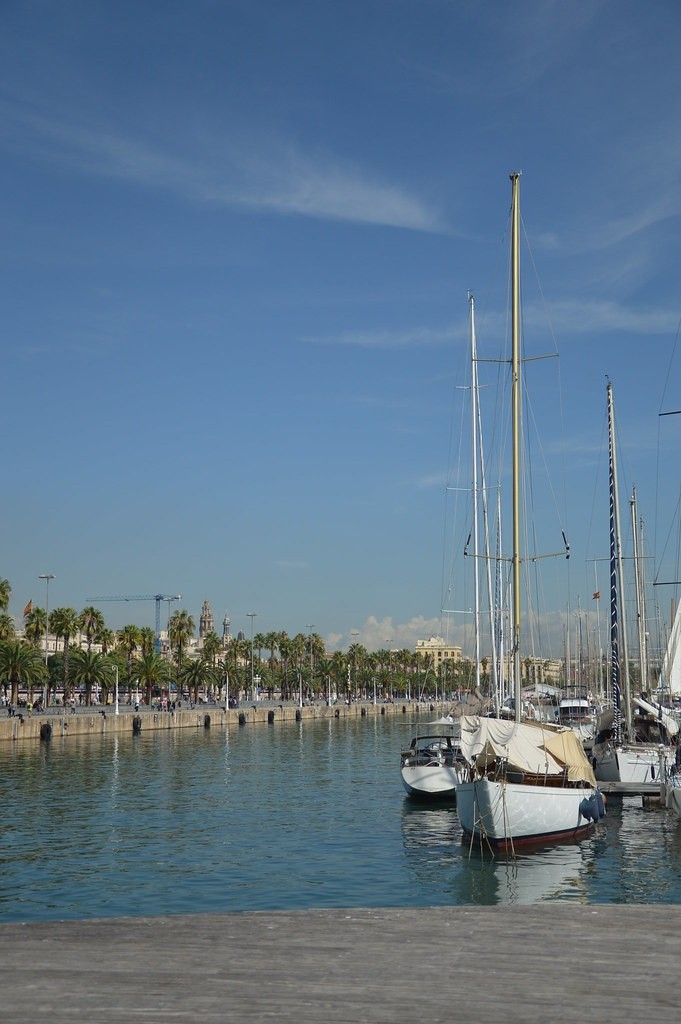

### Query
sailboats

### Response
[{"left": 403, "top": 173, "right": 681, "bottom": 867}]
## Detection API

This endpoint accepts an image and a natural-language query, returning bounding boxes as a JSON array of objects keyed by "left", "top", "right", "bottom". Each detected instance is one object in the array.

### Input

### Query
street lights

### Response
[
  {"left": 386, "top": 640, "right": 394, "bottom": 650},
  {"left": 38, "top": 574, "right": 57, "bottom": 710},
  {"left": 304, "top": 625, "right": 316, "bottom": 669},
  {"left": 247, "top": 612, "right": 258, "bottom": 699},
  {"left": 297, "top": 673, "right": 303, "bottom": 707},
  {"left": 326, "top": 675, "right": 331, "bottom": 706},
  {"left": 112, "top": 664, "right": 120, "bottom": 714},
  {"left": 351, "top": 633, "right": 360, "bottom": 644},
  {"left": 223, "top": 671, "right": 231, "bottom": 710}
]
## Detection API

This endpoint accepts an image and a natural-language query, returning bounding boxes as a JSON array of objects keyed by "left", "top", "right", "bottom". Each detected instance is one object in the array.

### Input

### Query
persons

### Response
[
  {"left": 198, "top": 696, "right": 209, "bottom": 704},
  {"left": 70, "top": 700, "right": 76, "bottom": 714},
  {"left": 281, "top": 691, "right": 289, "bottom": 702},
  {"left": 229, "top": 695, "right": 237, "bottom": 708},
  {"left": 78, "top": 692, "right": 85, "bottom": 706},
  {"left": 384, "top": 697, "right": 391, "bottom": 703},
  {"left": 351, "top": 696, "right": 357, "bottom": 702},
  {"left": 305, "top": 697, "right": 315, "bottom": 706},
  {"left": 0, "top": 692, "right": 56, "bottom": 718},
  {"left": 420, "top": 693, "right": 434, "bottom": 703},
  {"left": 150, "top": 697, "right": 183, "bottom": 712}
]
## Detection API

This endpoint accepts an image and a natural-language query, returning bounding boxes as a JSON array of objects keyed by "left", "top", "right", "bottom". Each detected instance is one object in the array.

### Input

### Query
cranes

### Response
[{"left": 84, "top": 594, "right": 182, "bottom": 639}]
